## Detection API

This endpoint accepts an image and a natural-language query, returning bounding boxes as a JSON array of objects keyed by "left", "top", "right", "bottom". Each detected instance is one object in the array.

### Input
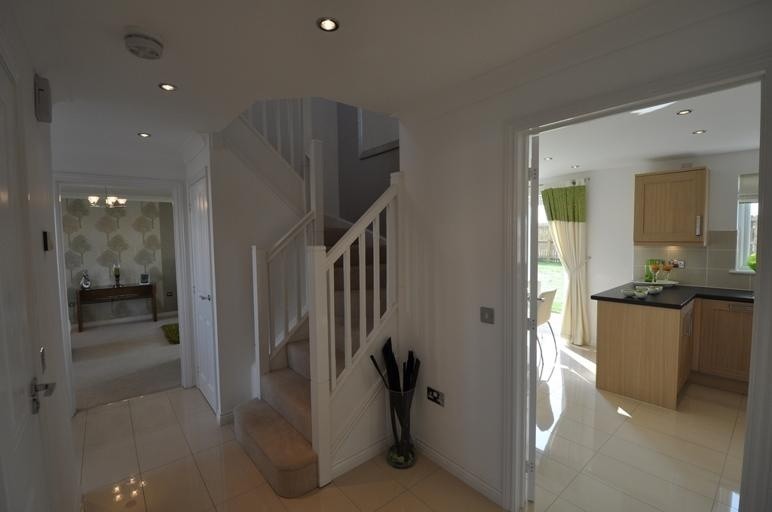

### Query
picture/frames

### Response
[{"left": 139, "top": 273, "right": 150, "bottom": 285}]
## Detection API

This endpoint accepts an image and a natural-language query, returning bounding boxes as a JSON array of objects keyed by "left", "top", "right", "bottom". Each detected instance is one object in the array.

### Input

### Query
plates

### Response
[{"left": 632, "top": 279, "right": 679, "bottom": 288}]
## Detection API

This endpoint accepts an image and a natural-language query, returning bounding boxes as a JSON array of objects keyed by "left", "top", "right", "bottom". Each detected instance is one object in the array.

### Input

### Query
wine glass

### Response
[
  {"left": 648, "top": 258, "right": 660, "bottom": 282},
  {"left": 661, "top": 258, "right": 673, "bottom": 282}
]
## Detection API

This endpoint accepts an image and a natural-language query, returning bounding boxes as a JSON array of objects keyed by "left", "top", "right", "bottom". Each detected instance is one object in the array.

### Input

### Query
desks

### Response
[{"left": 75, "top": 282, "right": 158, "bottom": 332}]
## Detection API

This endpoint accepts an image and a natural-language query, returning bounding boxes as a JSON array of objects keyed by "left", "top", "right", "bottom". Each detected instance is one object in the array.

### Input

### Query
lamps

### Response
[
  {"left": 88, "top": 185, "right": 127, "bottom": 208},
  {"left": 124, "top": 34, "right": 163, "bottom": 59}
]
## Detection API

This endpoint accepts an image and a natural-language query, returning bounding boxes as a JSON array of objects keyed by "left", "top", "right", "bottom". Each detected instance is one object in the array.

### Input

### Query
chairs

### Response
[{"left": 528, "top": 289, "right": 557, "bottom": 365}]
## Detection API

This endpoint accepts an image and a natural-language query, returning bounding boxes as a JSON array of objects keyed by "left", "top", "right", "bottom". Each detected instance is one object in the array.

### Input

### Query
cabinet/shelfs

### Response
[
  {"left": 595, "top": 297, "right": 753, "bottom": 411},
  {"left": 633, "top": 166, "right": 710, "bottom": 248}
]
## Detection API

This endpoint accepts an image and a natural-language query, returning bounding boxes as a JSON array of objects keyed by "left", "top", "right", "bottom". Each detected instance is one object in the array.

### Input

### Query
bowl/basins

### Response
[{"left": 621, "top": 285, "right": 664, "bottom": 299}]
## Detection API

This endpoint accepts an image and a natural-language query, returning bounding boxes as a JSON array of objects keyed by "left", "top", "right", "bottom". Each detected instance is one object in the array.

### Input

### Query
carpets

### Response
[{"left": 161, "top": 323, "right": 180, "bottom": 344}]
